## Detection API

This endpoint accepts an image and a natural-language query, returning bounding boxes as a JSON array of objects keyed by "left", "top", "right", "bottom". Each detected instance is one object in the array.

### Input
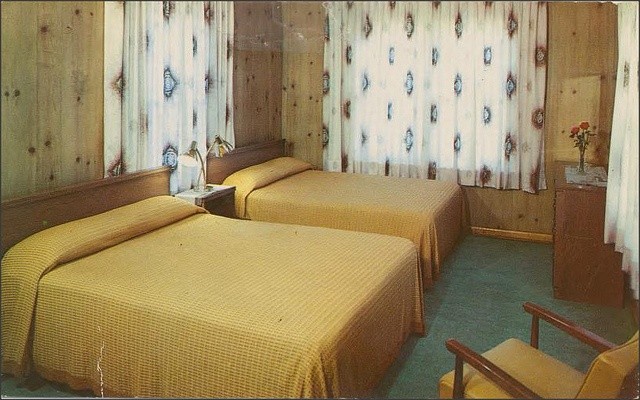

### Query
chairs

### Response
[{"left": 437, "top": 302, "right": 640, "bottom": 400}]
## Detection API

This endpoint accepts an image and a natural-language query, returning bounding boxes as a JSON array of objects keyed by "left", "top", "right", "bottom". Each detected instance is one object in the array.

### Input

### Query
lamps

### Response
[
  {"left": 177, "top": 140, "right": 216, "bottom": 192},
  {"left": 194, "top": 134, "right": 235, "bottom": 192}
]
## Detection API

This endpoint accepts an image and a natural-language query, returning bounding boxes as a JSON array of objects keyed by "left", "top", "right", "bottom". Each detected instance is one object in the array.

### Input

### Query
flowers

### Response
[{"left": 569, "top": 121, "right": 596, "bottom": 167}]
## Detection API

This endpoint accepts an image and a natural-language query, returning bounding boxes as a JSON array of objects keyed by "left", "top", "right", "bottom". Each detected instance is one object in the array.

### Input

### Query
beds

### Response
[
  {"left": 199, "top": 139, "right": 465, "bottom": 288},
  {"left": 0, "top": 165, "right": 428, "bottom": 400}
]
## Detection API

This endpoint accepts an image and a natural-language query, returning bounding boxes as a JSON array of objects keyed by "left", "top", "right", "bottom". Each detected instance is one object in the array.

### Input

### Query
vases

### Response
[{"left": 576, "top": 147, "right": 585, "bottom": 175}]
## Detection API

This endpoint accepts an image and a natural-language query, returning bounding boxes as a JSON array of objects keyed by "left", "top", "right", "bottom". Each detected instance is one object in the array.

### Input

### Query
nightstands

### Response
[{"left": 175, "top": 182, "right": 237, "bottom": 218}]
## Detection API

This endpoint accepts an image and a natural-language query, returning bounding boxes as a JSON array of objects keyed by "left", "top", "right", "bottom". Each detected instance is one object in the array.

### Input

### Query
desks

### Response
[{"left": 552, "top": 161, "right": 627, "bottom": 308}]
